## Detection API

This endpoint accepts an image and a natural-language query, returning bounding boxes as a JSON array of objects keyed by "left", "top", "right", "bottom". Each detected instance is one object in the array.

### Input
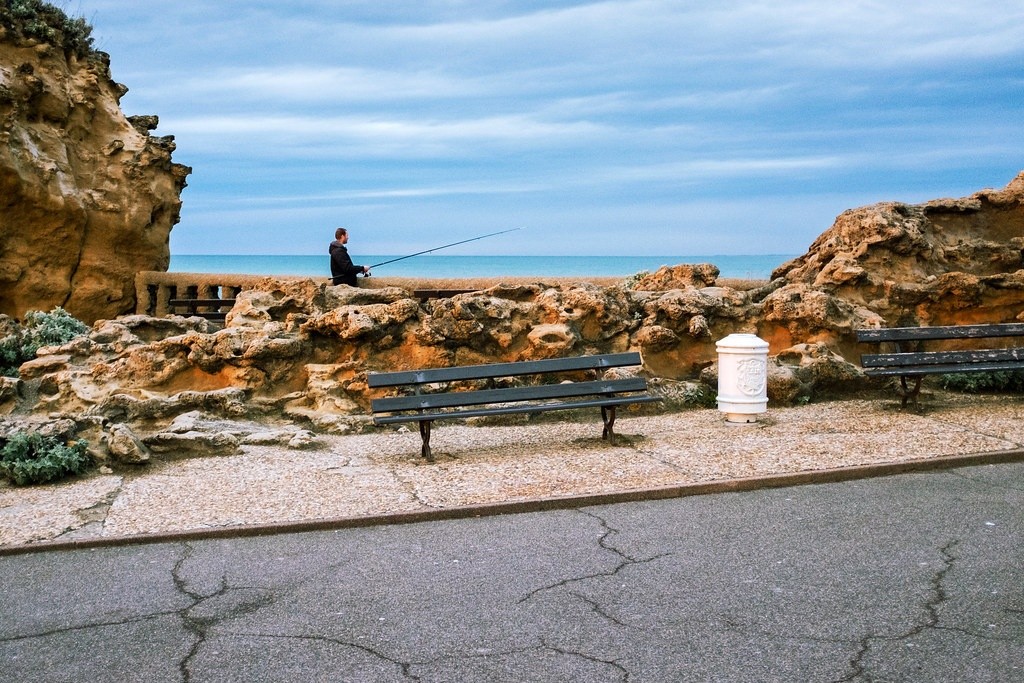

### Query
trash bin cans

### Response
[{"left": 714, "top": 333, "right": 770, "bottom": 423}]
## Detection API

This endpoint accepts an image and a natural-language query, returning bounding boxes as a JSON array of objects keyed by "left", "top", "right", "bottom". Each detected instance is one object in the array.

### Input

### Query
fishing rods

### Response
[{"left": 328, "top": 225, "right": 528, "bottom": 280}]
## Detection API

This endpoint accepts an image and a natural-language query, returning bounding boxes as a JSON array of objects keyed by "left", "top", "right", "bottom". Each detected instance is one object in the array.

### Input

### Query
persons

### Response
[{"left": 329, "top": 227, "right": 369, "bottom": 287}]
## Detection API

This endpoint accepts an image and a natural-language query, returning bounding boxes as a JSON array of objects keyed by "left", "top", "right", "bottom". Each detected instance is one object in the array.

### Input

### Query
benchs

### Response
[
  {"left": 368, "top": 353, "right": 663, "bottom": 463},
  {"left": 170, "top": 299, "right": 236, "bottom": 324},
  {"left": 414, "top": 290, "right": 479, "bottom": 303},
  {"left": 857, "top": 322, "right": 1024, "bottom": 412}
]
